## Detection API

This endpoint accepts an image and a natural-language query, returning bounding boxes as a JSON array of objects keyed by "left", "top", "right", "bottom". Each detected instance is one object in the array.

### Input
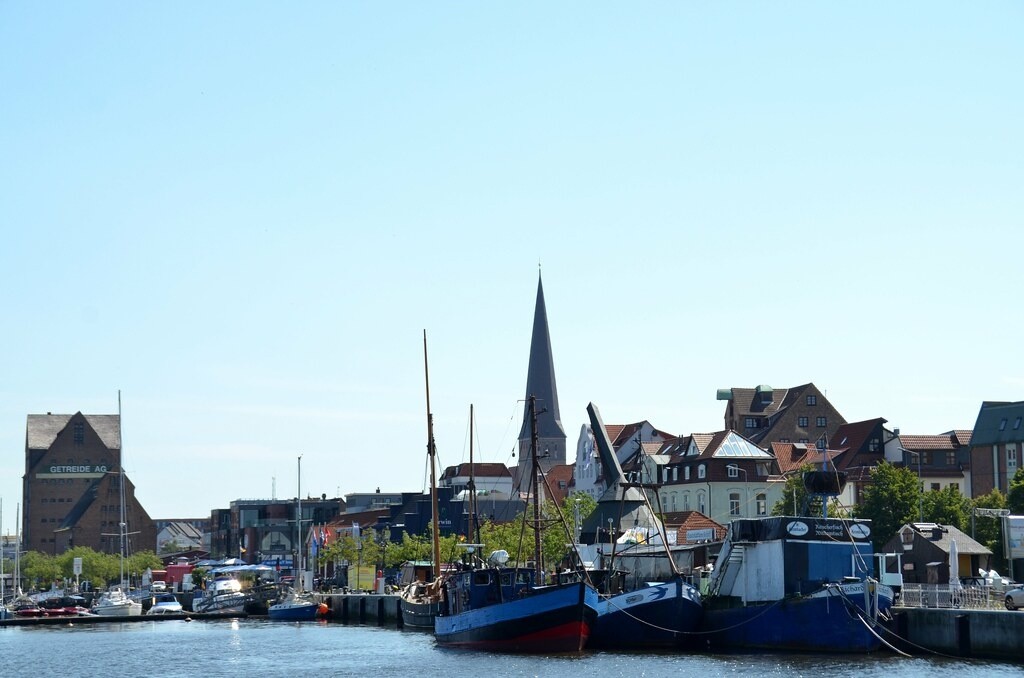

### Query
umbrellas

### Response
[
  {"left": 948, "top": 538, "right": 963, "bottom": 608},
  {"left": 276, "top": 557, "right": 280, "bottom": 577},
  {"left": 221, "top": 558, "right": 248, "bottom": 565},
  {"left": 194, "top": 559, "right": 220, "bottom": 565}
]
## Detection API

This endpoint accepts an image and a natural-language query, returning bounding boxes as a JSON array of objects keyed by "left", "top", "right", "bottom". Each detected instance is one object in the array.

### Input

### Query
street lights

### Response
[
  {"left": 726, "top": 463, "right": 750, "bottom": 518},
  {"left": 896, "top": 446, "right": 923, "bottom": 524}
]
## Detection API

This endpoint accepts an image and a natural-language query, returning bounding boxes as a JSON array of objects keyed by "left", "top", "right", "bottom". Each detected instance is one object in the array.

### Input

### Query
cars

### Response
[{"left": 1004, "top": 585, "right": 1024, "bottom": 610}]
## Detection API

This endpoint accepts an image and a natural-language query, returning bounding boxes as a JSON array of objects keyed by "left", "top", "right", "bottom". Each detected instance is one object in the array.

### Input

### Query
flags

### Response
[
  {"left": 324, "top": 527, "right": 331, "bottom": 546},
  {"left": 319, "top": 525, "right": 324, "bottom": 558},
  {"left": 312, "top": 526, "right": 317, "bottom": 557}
]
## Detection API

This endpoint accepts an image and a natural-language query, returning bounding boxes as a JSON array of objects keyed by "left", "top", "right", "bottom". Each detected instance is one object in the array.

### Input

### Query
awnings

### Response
[{"left": 207, "top": 564, "right": 273, "bottom": 573}]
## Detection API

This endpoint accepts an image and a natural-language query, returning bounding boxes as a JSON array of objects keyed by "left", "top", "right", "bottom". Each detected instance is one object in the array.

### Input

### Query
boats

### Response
[{"left": 0, "top": 255, "right": 1024, "bottom": 655}]
